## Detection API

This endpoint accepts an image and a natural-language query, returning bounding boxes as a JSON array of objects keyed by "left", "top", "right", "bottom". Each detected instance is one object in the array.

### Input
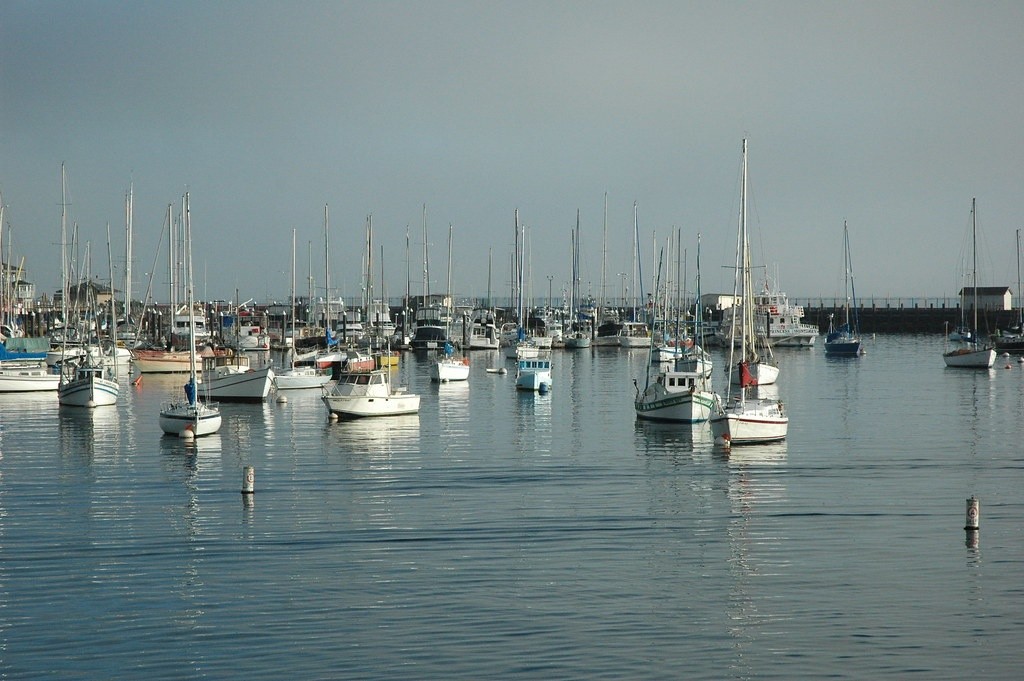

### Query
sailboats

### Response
[
  {"left": 709, "top": 135, "right": 789, "bottom": 445},
  {"left": 985, "top": 227, "right": 1024, "bottom": 356},
  {"left": 1, "top": 157, "right": 724, "bottom": 425},
  {"left": 160, "top": 188, "right": 222, "bottom": 438},
  {"left": 948, "top": 250, "right": 972, "bottom": 342},
  {"left": 943, "top": 196, "right": 998, "bottom": 369},
  {"left": 824, "top": 219, "right": 862, "bottom": 354}
]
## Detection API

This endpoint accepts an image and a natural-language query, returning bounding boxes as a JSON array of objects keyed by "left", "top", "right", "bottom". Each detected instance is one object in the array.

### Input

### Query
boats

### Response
[{"left": 712, "top": 264, "right": 820, "bottom": 349}]
